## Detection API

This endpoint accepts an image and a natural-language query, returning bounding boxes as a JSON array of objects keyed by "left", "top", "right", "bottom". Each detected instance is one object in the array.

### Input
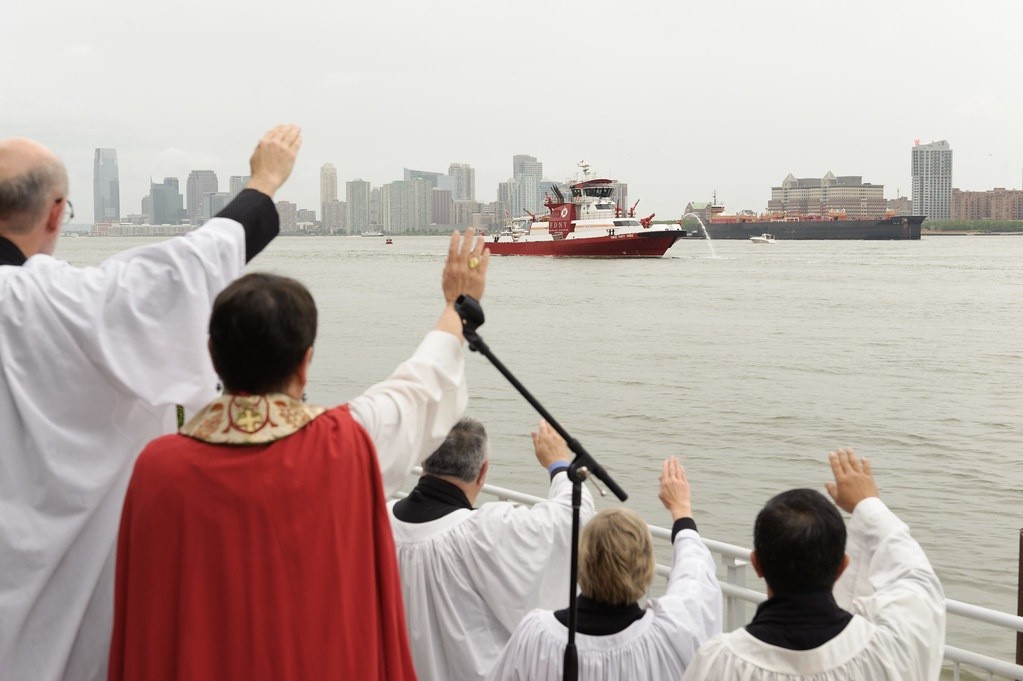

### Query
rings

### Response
[{"left": 468, "top": 257, "right": 480, "bottom": 269}]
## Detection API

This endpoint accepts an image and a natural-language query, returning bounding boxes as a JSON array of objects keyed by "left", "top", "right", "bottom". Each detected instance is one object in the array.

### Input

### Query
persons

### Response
[
  {"left": 385, "top": 418, "right": 595, "bottom": 681},
  {"left": 0, "top": 123, "right": 303, "bottom": 681},
  {"left": 106, "top": 227, "right": 491, "bottom": 681},
  {"left": 485, "top": 455, "right": 723, "bottom": 681},
  {"left": 679, "top": 446, "right": 946, "bottom": 681}
]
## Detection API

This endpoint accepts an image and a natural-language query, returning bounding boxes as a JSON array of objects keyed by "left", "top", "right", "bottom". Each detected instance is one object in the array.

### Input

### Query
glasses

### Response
[{"left": 54, "top": 197, "right": 74, "bottom": 223}]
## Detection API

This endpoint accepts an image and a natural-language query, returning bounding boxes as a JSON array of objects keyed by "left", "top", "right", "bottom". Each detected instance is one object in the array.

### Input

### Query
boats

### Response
[
  {"left": 678, "top": 189, "right": 929, "bottom": 242},
  {"left": 360, "top": 231, "right": 384, "bottom": 238},
  {"left": 483, "top": 159, "right": 686, "bottom": 259},
  {"left": 750, "top": 233, "right": 776, "bottom": 244}
]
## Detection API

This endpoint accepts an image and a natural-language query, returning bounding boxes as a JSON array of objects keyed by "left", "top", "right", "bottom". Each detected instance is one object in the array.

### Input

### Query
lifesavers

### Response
[{"left": 560, "top": 208, "right": 568, "bottom": 218}]
